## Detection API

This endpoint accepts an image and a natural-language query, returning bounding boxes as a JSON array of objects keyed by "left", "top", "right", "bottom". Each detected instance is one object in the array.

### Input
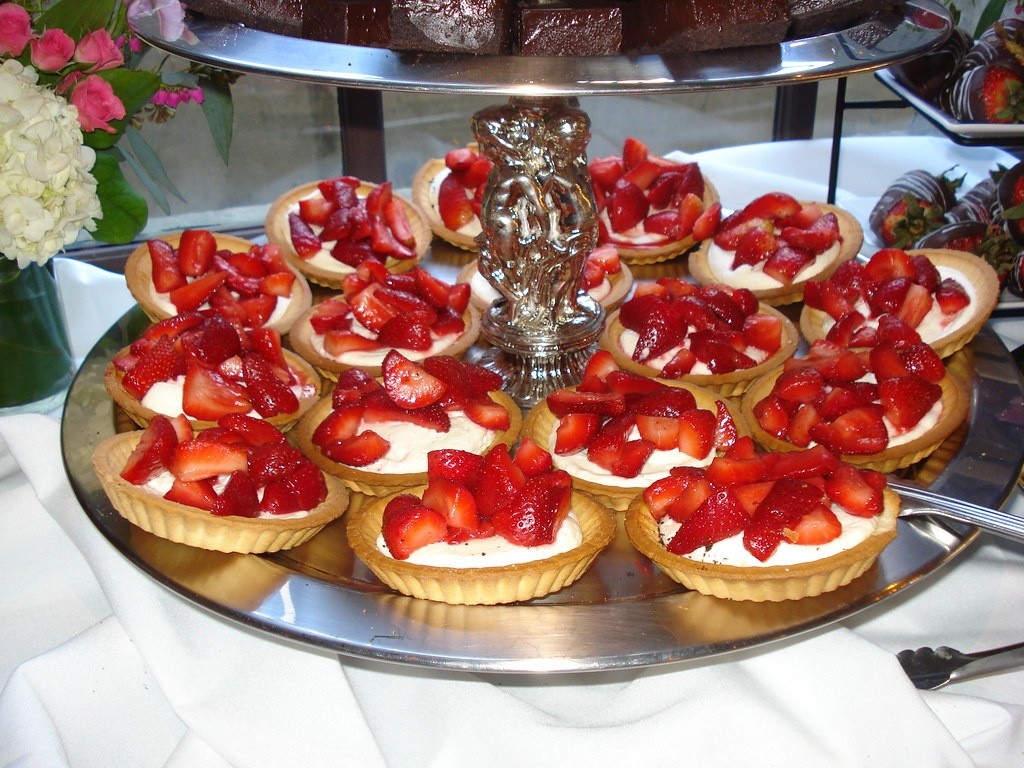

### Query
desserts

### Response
[
  {"left": 868, "top": 156, "right": 1024, "bottom": 304},
  {"left": 886, "top": 16, "right": 1024, "bottom": 128},
  {"left": 91, "top": 139, "right": 999, "bottom": 604}
]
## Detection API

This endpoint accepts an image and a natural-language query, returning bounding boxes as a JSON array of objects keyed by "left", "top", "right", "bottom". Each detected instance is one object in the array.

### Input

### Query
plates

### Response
[
  {"left": 838, "top": 197, "right": 1024, "bottom": 309},
  {"left": 876, "top": 65, "right": 1024, "bottom": 136}
]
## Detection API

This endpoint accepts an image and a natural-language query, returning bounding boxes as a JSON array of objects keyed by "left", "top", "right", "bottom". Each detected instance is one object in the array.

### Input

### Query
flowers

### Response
[{"left": 0, "top": 0, "right": 245, "bottom": 411}]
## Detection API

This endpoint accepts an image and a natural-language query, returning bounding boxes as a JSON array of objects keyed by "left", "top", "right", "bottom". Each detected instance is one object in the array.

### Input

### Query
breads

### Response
[{"left": 184, "top": 0, "right": 908, "bottom": 59}]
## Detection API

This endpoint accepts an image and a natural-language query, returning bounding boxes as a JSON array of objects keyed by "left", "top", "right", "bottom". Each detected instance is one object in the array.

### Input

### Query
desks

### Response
[{"left": 0, "top": 133, "right": 1024, "bottom": 768}]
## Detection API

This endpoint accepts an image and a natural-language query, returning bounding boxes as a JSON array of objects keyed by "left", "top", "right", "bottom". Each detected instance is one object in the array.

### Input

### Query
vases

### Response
[{"left": 0, "top": 251, "right": 77, "bottom": 417}]
